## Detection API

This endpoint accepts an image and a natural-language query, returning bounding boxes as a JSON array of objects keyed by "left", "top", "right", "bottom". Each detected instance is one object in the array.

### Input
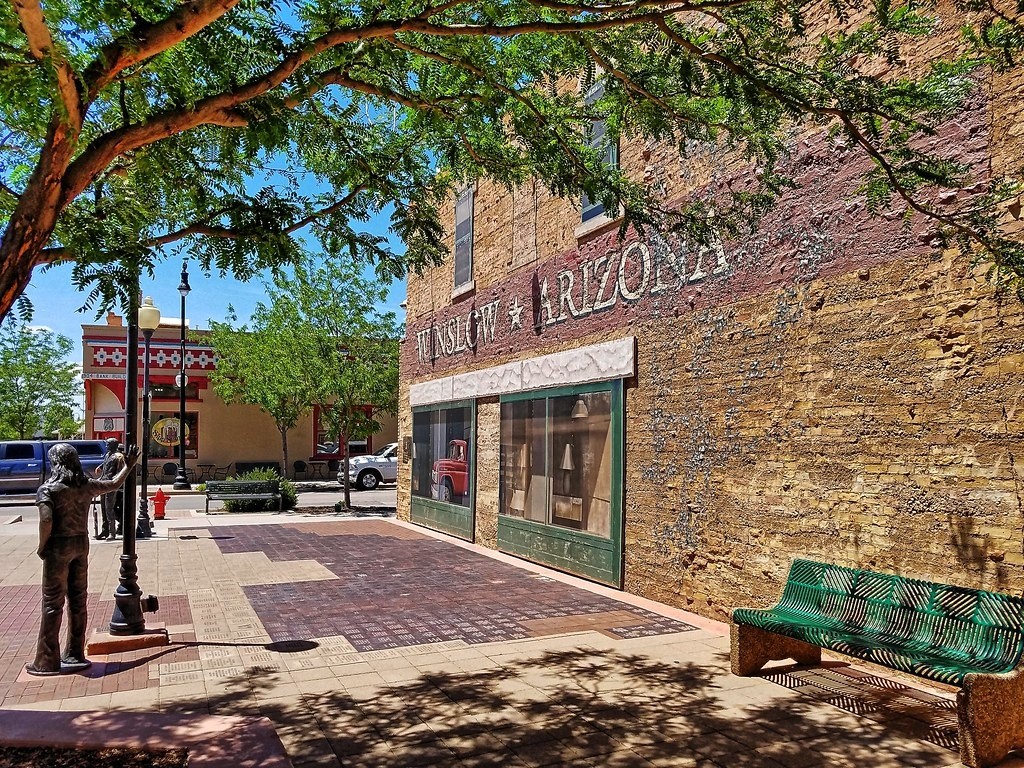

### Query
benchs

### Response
[
  {"left": 729, "top": 558, "right": 1024, "bottom": 768},
  {"left": 235, "top": 462, "right": 283, "bottom": 482},
  {"left": 204, "top": 481, "right": 283, "bottom": 512}
]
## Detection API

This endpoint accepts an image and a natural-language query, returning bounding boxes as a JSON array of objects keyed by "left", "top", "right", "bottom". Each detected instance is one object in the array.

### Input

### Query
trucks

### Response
[{"left": 431, "top": 440, "right": 469, "bottom": 503}]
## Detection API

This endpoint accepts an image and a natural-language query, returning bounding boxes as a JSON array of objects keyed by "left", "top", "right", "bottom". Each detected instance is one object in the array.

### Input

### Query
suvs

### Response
[{"left": 337, "top": 442, "right": 398, "bottom": 490}]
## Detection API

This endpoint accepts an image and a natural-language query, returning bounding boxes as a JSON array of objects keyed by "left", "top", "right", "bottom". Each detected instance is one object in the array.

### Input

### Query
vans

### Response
[{"left": 1, "top": 439, "right": 110, "bottom": 499}]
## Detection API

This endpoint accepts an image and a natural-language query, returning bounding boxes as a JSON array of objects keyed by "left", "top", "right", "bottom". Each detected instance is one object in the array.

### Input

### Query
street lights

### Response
[
  {"left": 173, "top": 257, "right": 193, "bottom": 492},
  {"left": 175, "top": 370, "right": 189, "bottom": 487},
  {"left": 136, "top": 296, "right": 161, "bottom": 539}
]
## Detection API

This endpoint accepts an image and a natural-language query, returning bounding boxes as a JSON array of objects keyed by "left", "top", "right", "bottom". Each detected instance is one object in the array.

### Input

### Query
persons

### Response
[
  {"left": 95, "top": 438, "right": 125, "bottom": 541},
  {"left": 113, "top": 443, "right": 124, "bottom": 535},
  {"left": 25, "top": 444, "right": 142, "bottom": 671}
]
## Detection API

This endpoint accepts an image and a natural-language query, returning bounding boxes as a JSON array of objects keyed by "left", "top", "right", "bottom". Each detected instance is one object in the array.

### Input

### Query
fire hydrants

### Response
[{"left": 149, "top": 488, "right": 171, "bottom": 520}]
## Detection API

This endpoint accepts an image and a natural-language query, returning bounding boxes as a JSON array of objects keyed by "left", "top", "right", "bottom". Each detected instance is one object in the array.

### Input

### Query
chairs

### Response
[
  {"left": 161, "top": 462, "right": 178, "bottom": 484},
  {"left": 291, "top": 461, "right": 309, "bottom": 481},
  {"left": 327, "top": 459, "right": 342, "bottom": 481},
  {"left": 214, "top": 463, "right": 232, "bottom": 481}
]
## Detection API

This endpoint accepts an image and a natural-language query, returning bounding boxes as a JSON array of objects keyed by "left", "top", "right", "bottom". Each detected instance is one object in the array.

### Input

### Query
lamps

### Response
[
  {"left": 571, "top": 400, "right": 588, "bottom": 418},
  {"left": 559, "top": 444, "right": 575, "bottom": 471}
]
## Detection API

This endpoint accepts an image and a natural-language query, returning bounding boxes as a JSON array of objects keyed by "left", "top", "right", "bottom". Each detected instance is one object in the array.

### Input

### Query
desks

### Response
[
  {"left": 309, "top": 462, "right": 325, "bottom": 481},
  {"left": 510, "top": 490, "right": 583, "bottom": 521},
  {"left": 197, "top": 464, "right": 216, "bottom": 483}
]
